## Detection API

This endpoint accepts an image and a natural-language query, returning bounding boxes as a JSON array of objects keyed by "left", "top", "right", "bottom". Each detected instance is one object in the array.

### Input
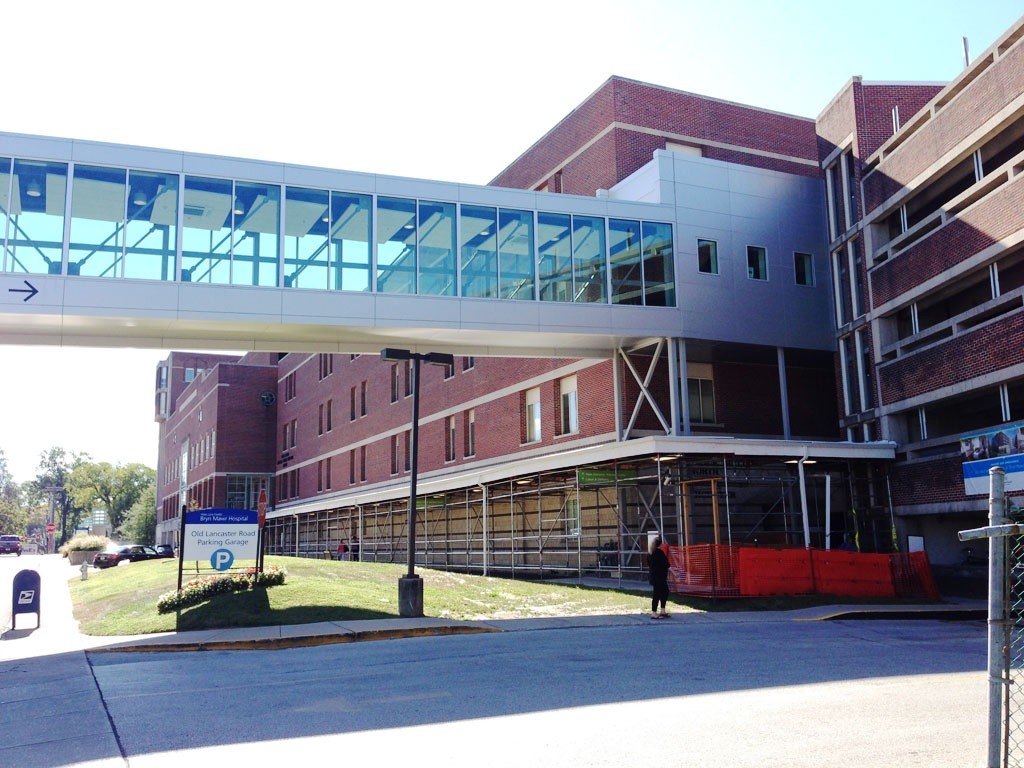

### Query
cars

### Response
[{"left": 93, "top": 545, "right": 168, "bottom": 569}]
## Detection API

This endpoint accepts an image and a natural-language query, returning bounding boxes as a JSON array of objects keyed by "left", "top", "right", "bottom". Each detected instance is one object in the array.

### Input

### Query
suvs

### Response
[
  {"left": 0, "top": 535, "right": 22, "bottom": 556},
  {"left": 154, "top": 544, "right": 173, "bottom": 558}
]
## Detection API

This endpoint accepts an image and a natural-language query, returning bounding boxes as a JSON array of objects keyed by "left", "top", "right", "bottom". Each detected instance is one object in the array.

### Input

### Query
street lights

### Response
[{"left": 381, "top": 348, "right": 453, "bottom": 616}]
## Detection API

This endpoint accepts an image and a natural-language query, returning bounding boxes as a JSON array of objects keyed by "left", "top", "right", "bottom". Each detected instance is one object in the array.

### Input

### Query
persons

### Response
[
  {"left": 337, "top": 536, "right": 359, "bottom": 562},
  {"left": 840, "top": 536, "right": 858, "bottom": 552},
  {"left": 646, "top": 537, "right": 670, "bottom": 620}
]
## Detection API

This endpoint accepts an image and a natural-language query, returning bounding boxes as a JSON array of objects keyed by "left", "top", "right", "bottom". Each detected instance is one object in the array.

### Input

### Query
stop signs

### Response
[
  {"left": 257, "top": 489, "right": 267, "bottom": 529},
  {"left": 47, "top": 523, "right": 56, "bottom": 532}
]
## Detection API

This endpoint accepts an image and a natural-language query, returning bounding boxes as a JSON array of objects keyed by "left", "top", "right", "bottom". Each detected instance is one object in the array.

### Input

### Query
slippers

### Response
[
  {"left": 660, "top": 613, "right": 671, "bottom": 617},
  {"left": 651, "top": 615, "right": 664, "bottom": 619}
]
